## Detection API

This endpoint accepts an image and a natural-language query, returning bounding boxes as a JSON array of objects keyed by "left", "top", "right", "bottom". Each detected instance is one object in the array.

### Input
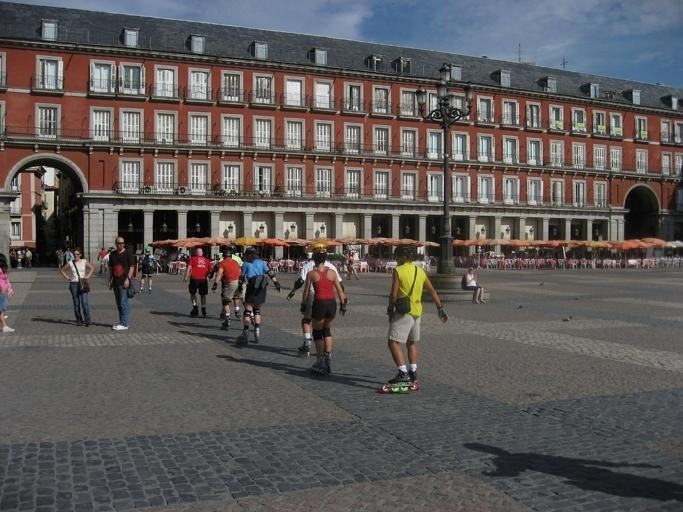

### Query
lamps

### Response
[
  {"left": 405, "top": 226, "right": 409, "bottom": 234},
  {"left": 595, "top": 229, "right": 598, "bottom": 234},
  {"left": 195, "top": 223, "right": 201, "bottom": 232},
  {"left": 432, "top": 226, "right": 436, "bottom": 233},
  {"left": 481, "top": 227, "right": 485, "bottom": 234},
  {"left": 290, "top": 223, "right": 296, "bottom": 232},
  {"left": 529, "top": 227, "right": 534, "bottom": 234},
  {"left": 161, "top": 223, "right": 168, "bottom": 233},
  {"left": 377, "top": 225, "right": 382, "bottom": 234},
  {"left": 259, "top": 223, "right": 265, "bottom": 232},
  {"left": 575, "top": 229, "right": 578, "bottom": 234},
  {"left": 553, "top": 228, "right": 557, "bottom": 234},
  {"left": 227, "top": 223, "right": 233, "bottom": 233},
  {"left": 320, "top": 224, "right": 325, "bottom": 233},
  {"left": 456, "top": 227, "right": 460, "bottom": 234},
  {"left": 506, "top": 226, "right": 510, "bottom": 234},
  {"left": 128, "top": 223, "right": 133, "bottom": 233}
]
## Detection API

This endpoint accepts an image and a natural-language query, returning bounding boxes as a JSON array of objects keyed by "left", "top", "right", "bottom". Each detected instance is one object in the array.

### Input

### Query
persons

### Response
[
  {"left": 57, "top": 237, "right": 163, "bottom": 330},
  {"left": 381, "top": 246, "right": 449, "bottom": 392},
  {"left": 175, "top": 249, "right": 439, "bottom": 280},
  {"left": 0, "top": 253, "right": 16, "bottom": 331},
  {"left": 159, "top": 254, "right": 169, "bottom": 271},
  {"left": 169, "top": 251, "right": 179, "bottom": 262},
  {"left": 286, "top": 240, "right": 347, "bottom": 376},
  {"left": 461, "top": 267, "right": 485, "bottom": 304},
  {"left": 10, "top": 247, "right": 33, "bottom": 268},
  {"left": 185, "top": 248, "right": 280, "bottom": 346}
]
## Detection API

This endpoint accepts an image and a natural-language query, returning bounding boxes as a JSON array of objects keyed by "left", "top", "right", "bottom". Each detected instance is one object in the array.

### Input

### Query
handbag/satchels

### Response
[
  {"left": 77, "top": 277, "right": 90, "bottom": 293},
  {"left": 127, "top": 276, "right": 136, "bottom": 298},
  {"left": 395, "top": 296, "right": 411, "bottom": 315}
]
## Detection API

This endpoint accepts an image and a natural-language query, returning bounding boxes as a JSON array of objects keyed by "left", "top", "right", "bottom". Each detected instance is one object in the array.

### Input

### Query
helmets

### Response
[
  {"left": 244, "top": 247, "right": 257, "bottom": 255},
  {"left": 310, "top": 241, "right": 326, "bottom": 249},
  {"left": 222, "top": 250, "right": 232, "bottom": 257},
  {"left": 145, "top": 247, "right": 151, "bottom": 252},
  {"left": 394, "top": 244, "right": 416, "bottom": 255}
]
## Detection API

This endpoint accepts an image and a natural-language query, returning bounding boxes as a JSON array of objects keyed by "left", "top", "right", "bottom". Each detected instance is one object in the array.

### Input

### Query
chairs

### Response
[
  {"left": 458, "top": 254, "right": 683, "bottom": 270},
  {"left": 147, "top": 252, "right": 432, "bottom": 277}
]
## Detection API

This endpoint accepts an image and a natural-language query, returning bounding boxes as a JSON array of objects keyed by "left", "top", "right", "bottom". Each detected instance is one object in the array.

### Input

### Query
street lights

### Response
[{"left": 414, "top": 58, "right": 475, "bottom": 290}]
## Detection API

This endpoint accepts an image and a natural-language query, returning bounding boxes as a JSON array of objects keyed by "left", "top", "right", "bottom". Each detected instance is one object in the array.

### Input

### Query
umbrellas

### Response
[{"left": 452, "top": 238, "right": 683, "bottom": 259}]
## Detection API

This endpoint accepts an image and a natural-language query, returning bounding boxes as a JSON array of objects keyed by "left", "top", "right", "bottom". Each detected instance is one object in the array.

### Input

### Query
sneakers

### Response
[
  {"left": 85, "top": 321, "right": 92, "bottom": 327},
  {"left": 112, "top": 322, "right": 129, "bottom": 331},
  {"left": 2, "top": 326, "right": 15, "bottom": 333},
  {"left": 76, "top": 319, "right": 83, "bottom": 326}
]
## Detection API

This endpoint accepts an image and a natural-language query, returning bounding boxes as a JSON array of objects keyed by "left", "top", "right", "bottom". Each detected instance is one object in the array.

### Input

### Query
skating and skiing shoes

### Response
[
  {"left": 236, "top": 329, "right": 249, "bottom": 346},
  {"left": 312, "top": 351, "right": 333, "bottom": 375},
  {"left": 297, "top": 338, "right": 313, "bottom": 359},
  {"left": 234, "top": 310, "right": 241, "bottom": 320},
  {"left": 382, "top": 369, "right": 419, "bottom": 392},
  {"left": 221, "top": 318, "right": 231, "bottom": 331},
  {"left": 201, "top": 306, "right": 207, "bottom": 317},
  {"left": 254, "top": 327, "right": 261, "bottom": 344},
  {"left": 190, "top": 305, "right": 199, "bottom": 318}
]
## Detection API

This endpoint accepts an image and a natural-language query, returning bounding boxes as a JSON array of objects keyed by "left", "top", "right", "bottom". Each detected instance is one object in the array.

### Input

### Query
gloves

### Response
[
  {"left": 238, "top": 278, "right": 245, "bottom": 294},
  {"left": 386, "top": 307, "right": 395, "bottom": 322},
  {"left": 286, "top": 288, "right": 296, "bottom": 302},
  {"left": 211, "top": 282, "right": 218, "bottom": 293},
  {"left": 273, "top": 281, "right": 282, "bottom": 294},
  {"left": 339, "top": 299, "right": 348, "bottom": 317},
  {"left": 436, "top": 303, "right": 449, "bottom": 324}
]
